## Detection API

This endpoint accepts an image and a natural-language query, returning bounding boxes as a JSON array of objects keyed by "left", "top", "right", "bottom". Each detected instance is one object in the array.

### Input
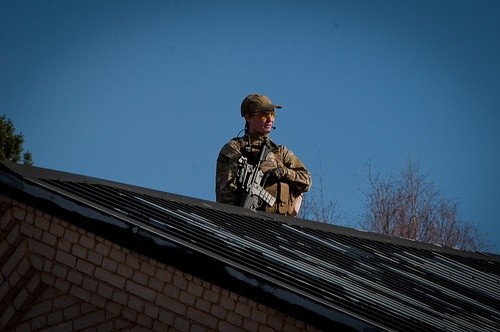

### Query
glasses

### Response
[{"left": 253, "top": 111, "right": 276, "bottom": 119}]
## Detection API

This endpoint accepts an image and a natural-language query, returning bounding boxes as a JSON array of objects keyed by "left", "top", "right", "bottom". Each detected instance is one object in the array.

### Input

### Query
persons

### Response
[{"left": 215, "top": 94, "right": 311, "bottom": 218}]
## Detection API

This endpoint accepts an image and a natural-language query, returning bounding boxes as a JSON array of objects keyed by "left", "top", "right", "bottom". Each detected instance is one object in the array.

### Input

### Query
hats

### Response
[{"left": 241, "top": 94, "right": 282, "bottom": 116}]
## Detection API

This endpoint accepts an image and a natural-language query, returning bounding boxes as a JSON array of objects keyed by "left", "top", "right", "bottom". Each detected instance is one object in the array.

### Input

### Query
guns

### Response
[{"left": 237, "top": 137, "right": 276, "bottom": 210}]
